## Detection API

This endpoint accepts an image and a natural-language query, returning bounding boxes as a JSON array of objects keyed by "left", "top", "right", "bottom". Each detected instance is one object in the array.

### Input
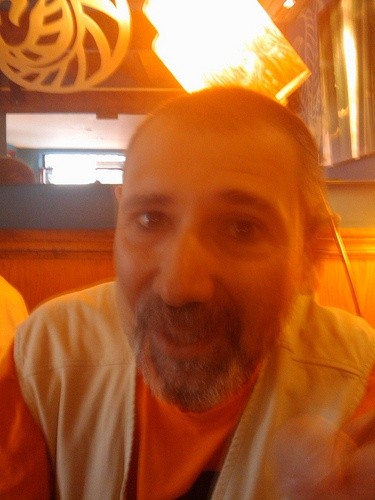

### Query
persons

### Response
[{"left": 0, "top": 88, "right": 375, "bottom": 500}]
